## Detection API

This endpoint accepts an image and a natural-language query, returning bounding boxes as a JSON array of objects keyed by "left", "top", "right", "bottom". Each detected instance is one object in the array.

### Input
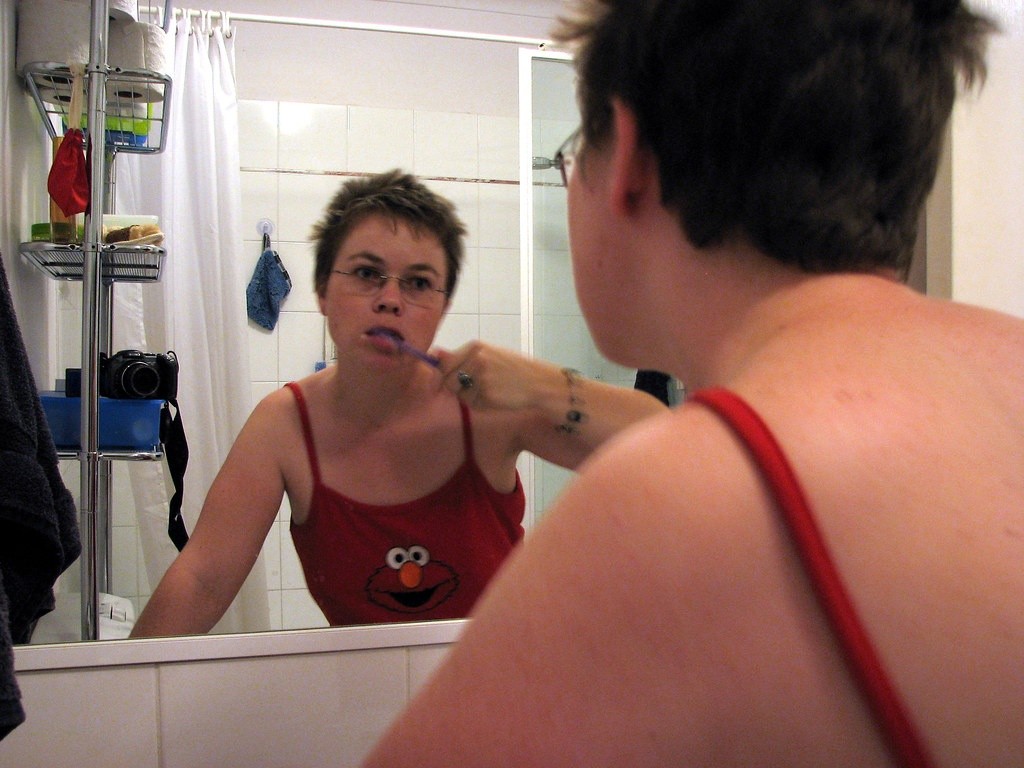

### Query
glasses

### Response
[
  {"left": 550, "top": 123, "right": 588, "bottom": 189},
  {"left": 329, "top": 264, "right": 448, "bottom": 311}
]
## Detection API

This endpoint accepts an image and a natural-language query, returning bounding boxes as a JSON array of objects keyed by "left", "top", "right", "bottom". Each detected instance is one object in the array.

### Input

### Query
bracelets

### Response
[{"left": 554, "top": 368, "right": 580, "bottom": 434}]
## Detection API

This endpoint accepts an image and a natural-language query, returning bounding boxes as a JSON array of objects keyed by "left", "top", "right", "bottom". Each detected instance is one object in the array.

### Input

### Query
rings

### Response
[{"left": 456, "top": 369, "right": 473, "bottom": 388}]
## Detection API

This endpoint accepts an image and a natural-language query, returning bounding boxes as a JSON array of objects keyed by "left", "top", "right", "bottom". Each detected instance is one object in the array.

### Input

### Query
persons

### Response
[
  {"left": 366, "top": 1, "right": 1023, "bottom": 768},
  {"left": 132, "top": 171, "right": 670, "bottom": 638}
]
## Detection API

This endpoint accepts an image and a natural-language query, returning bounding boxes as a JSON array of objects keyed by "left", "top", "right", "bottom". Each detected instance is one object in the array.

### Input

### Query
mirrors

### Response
[{"left": 0, "top": 6, "right": 687, "bottom": 670}]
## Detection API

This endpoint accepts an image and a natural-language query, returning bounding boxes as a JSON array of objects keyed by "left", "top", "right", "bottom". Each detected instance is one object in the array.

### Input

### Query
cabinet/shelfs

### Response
[{"left": 18, "top": 1, "right": 174, "bottom": 640}]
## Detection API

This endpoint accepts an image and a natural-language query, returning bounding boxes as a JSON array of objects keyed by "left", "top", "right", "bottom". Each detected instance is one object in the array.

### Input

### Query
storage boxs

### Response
[{"left": 38, "top": 390, "right": 166, "bottom": 447}]
